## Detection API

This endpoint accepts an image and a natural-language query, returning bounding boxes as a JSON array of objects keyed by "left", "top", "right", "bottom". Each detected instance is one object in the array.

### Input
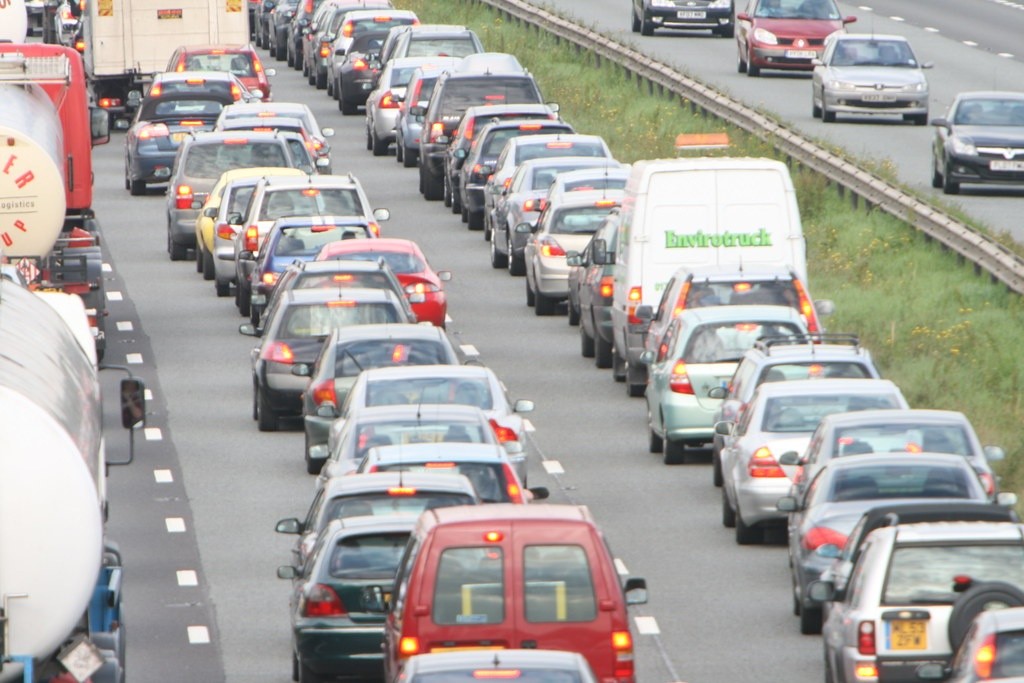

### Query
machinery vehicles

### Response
[
  {"left": 0, "top": 40, "right": 108, "bottom": 369},
  {"left": 0, "top": 270, "right": 147, "bottom": 683}
]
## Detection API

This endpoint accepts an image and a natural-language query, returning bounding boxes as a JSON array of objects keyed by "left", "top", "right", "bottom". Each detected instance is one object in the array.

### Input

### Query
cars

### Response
[
  {"left": 810, "top": 33, "right": 934, "bottom": 126},
  {"left": 931, "top": 89, "right": 1024, "bottom": 196},
  {"left": 632, "top": 0, "right": 735, "bottom": 39},
  {"left": 393, "top": 650, "right": 598, "bottom": 683},
  {"left": 734, "top": 0, "right": 858, "bottom": 77},
  {"left": 113, "top": 0, "right": 1023, "bottom": 683}
]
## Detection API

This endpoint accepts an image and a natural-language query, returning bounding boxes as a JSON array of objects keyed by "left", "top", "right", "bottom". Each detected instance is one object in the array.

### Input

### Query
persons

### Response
[
  {"left": 844, "top": 48, "right": 868, "bottom": 62},
  {"left": 761, "top": 0, "right": 786, "bottom": 15}
]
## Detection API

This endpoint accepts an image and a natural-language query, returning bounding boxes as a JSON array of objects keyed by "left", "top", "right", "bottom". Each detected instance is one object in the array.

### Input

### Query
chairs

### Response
[
  {"left": 443, "top": 424, "right": 470, "bottom": 441},
  {"left": 798, "top": 1, "right": 822, "bottom": 14},
  {"left": 692, "top": 287, "right": 720, "bottom": 307},
  {"left": 696, "top": 332, "right": 724, "bottom": 363},
  {"left": 340, "top": 554, "right": 367, "bottom": 568},
  {"left": 355, "top": 24, "right": 368, "bottom": 33},
  {"left": 842, "top": 439, "right": 874, "bottom": 455},
  {"left": 232, "top": 58, "right": 248, "bottom": 72},
  {"left": 456, "top": 383, "right": 478, "bottom": 410},
  {"left": 283, "top": 239, "right": 303, "bottom": 251},
  {"left": 842, "top": 47, "right": 856, "bottom": 57},
  {"left": 270, "top": 191, "right": 293, "bottom": 213},
  {"left": 493, "top": 137, "right": 505, "bottom": 150},
  {"left": 454, "top": 461, "right": 496, "bottom": 498},
  {"left": 365, "top": 434, "right": 393, "bottom": 448},
  {"left": 157, "top": 102, "right": 176, "bottom": 114},
  {"left": 343, "top": 502, "right": 373, "bottom": 515},
  {"left": 924, "top": 433, "right": 956, "bottom": 454},
  {"left": 925, "top": 468, "right": 958, "bottom": 494},
  {"left": 323, "top": 193, "right": 346, "bottom": 214},
  {"left": 880, "top": 46, "right": 899, "bottom": 61},
  {"left": 370, "top": 40, "right": 380, "bottom": 47},
  {"left": 775, "top": 409, "right": 803, "bottom": 429},
  {"left": 248, "top": 145, "right": 281, "bottom": 167},
  {"left": 842, "top": 475, "right": 878, "bottom": 499},
  {"left": 848, "top": 398, "right": 883, "bottom": 412},
  {"left": 193, "top": 145, "right": 222, "bottom": 180},
  {"left": 963, "top": 105, "right": 983, "bottom": 117},
  {"left": 827, "top": 366, "right": 861, "bottom": 379},
  {"left": 203, "top": 103, "right": 220, "bottom": 113},
  {"left": 766, "top": 370, "right": 784, "bottom": 382},
  {"left": 1011, "top": 106, "right": 1024, "bottom": 123},
  {"left": 575, "top": 147, "right": 592, "bottom": 157},
  {"left": 536, "top": 173, "right": 552, "bottom": 186}
]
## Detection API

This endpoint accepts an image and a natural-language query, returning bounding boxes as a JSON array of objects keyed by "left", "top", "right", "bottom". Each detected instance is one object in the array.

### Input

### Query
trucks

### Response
[{"left": 73, "top": 0, "right": 250, "bottom": 131}]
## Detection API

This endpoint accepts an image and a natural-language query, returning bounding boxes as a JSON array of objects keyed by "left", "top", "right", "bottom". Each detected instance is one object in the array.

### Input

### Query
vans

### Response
[
  {"left": 358, "top": 501, "right": 650, "bottom": 683},
  {"left": 592, "top": 155, "right": 810, "bottom": 398}
]
